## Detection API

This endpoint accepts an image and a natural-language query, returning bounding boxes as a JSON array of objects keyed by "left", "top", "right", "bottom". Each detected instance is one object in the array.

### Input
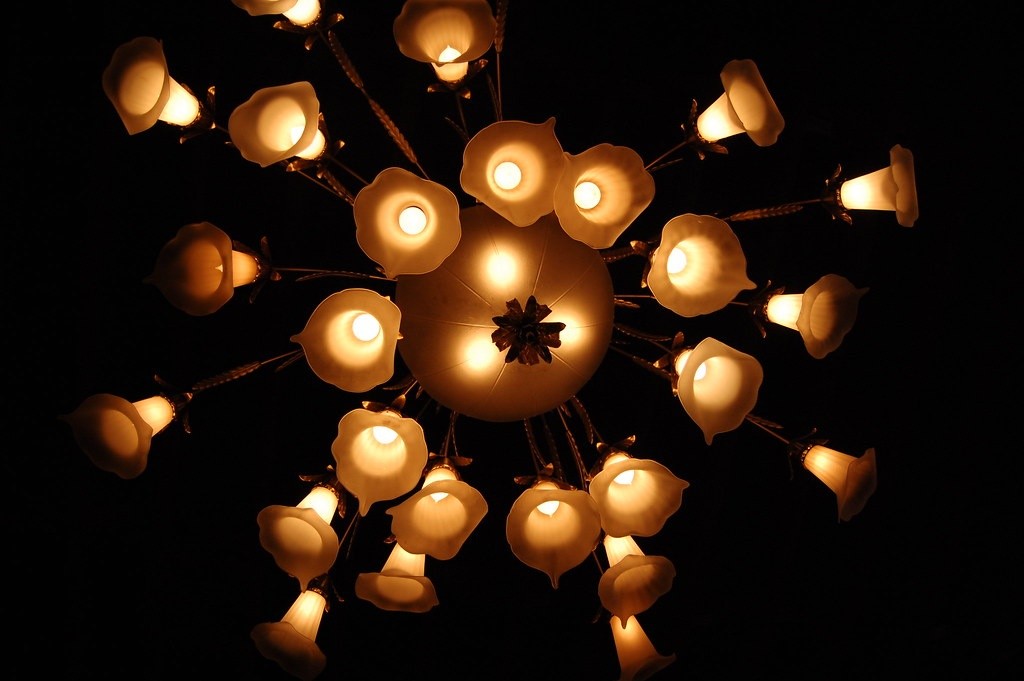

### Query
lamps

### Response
[{"left": 74, "top": 0, "right": 922, "bottom": 680}]
